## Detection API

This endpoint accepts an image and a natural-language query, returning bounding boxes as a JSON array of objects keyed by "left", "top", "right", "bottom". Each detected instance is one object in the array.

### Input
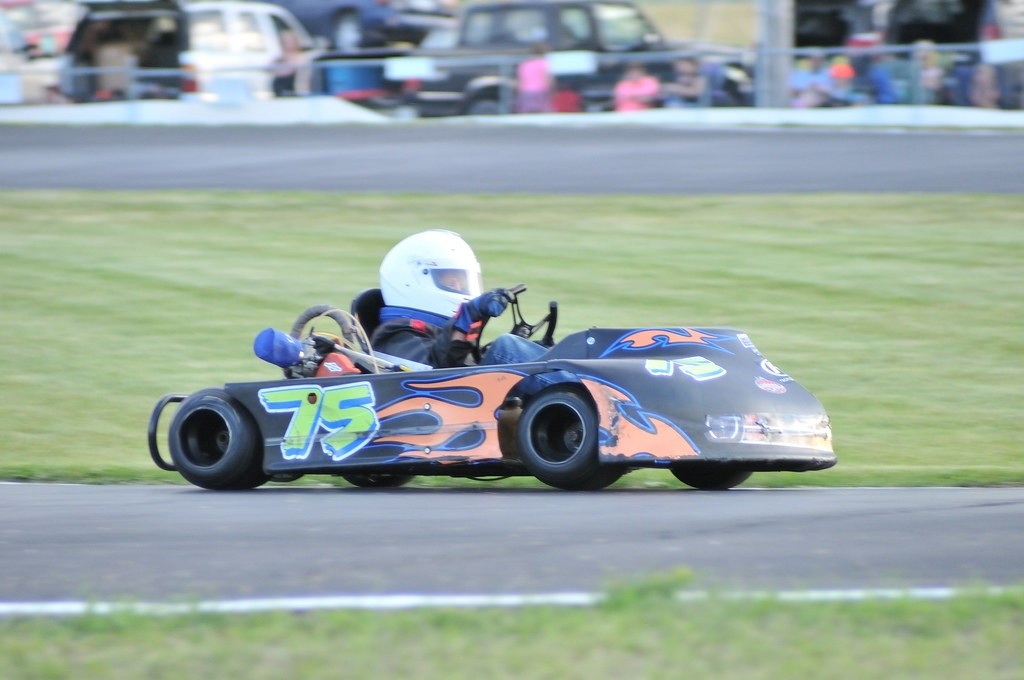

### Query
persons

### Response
[
  {"left": 779, "top": 41, "right": 833, "bottom": 108},
  {"left": 21, "top": 42, "right": 62, "bottom": 94},
  {"left": 664, "top": 50, "right": 708, "bottom": 108},
  {"left": 272, "top": 28, "right": 309, "bottom": 96},
  {"left": 614, "top": 65, "right": 659, "bottom": 112},
  {"left": 369, "top": 226, "right": 550, "bottom": 373},
  {"left": 516, "top": 44, "right": 554, "bottom": 116}
]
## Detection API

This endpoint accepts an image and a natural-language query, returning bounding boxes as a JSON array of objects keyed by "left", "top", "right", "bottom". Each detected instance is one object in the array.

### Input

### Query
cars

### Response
[{"left": 378, "top": 1, "right": 675, "bottom": 114}]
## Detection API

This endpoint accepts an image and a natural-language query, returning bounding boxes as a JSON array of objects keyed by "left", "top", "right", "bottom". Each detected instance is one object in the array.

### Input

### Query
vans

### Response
[{"left": 60, "top": 0, "right": 318, "bottom": 98}]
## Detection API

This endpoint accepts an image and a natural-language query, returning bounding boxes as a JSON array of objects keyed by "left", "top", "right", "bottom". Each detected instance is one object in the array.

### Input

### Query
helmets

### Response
[{"left": 378, "top": 229, "right": 481, "bottom": 331}]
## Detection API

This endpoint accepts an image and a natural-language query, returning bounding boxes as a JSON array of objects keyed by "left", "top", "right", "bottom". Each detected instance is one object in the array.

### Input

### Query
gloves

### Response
[{"left": 450, "top": 288, "right": 515, "bottom": 335}]
